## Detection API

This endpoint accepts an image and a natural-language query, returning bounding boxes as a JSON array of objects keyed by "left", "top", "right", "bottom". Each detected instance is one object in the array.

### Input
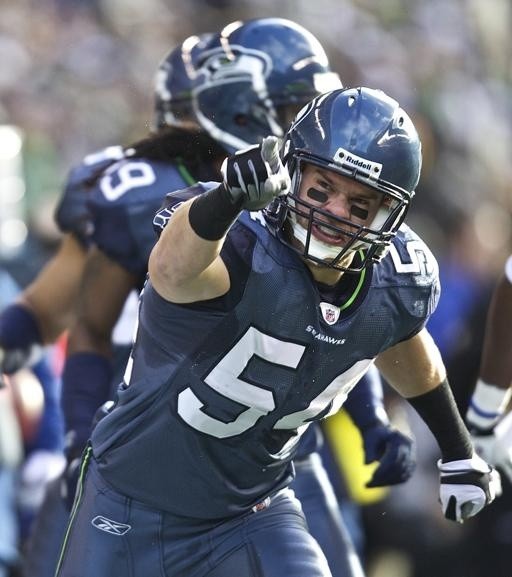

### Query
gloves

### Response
[
  {"left": 363, "top": 424, "right": 416, "bottom": 487},
  {"left": 220, "top": 136, "right": 292, "bottom": 211},
  {"left": 60, "top": 456, "right": 81, "bottom": 510},
  {"left": 436, "top": 420, "right": 494, "bottom": 524}
]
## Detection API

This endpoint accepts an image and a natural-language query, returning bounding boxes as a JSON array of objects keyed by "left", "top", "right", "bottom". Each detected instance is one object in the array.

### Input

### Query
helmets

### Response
[
  {"left": 263, "top": 85, "right": 423, "bottom": 274},
  {"left": 153, "top": 17, "right": 344, "bottom": 132}
]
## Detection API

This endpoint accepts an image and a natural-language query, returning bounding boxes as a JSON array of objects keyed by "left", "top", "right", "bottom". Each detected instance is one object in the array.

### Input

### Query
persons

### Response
[{"left": 0, "top": 1, "right": 512, "bottom": 577}]
